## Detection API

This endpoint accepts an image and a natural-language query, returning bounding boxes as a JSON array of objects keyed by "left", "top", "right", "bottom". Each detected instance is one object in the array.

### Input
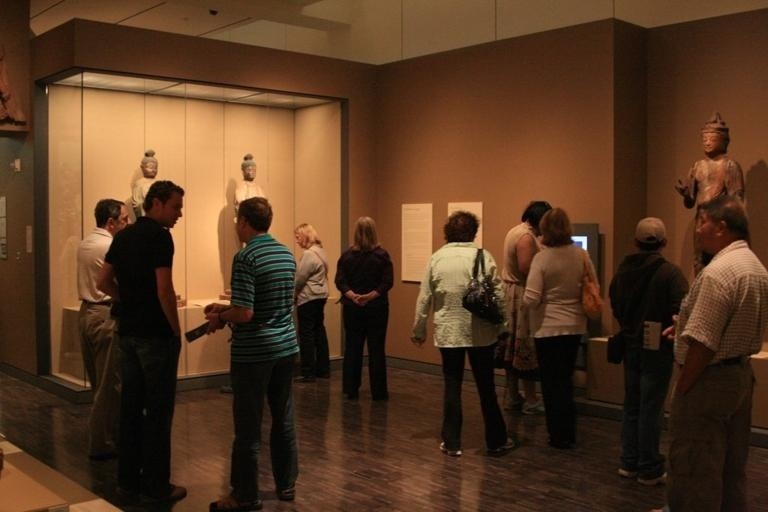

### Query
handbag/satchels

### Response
[
  {"left": 462, "top": 275, "right": 506, "bottom": 326},
  {"left": 581, "top": 274, "right": 605, "bottom": 322}
]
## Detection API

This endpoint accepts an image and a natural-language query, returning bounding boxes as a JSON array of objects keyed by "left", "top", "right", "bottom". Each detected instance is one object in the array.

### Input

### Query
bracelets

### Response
[{"left": 215, "top": 312, "right": 223, "bottom": 324}]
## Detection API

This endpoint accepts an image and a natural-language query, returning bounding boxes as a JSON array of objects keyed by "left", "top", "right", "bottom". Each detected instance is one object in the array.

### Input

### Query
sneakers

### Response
[
  {"left": 638, "top": 472, "right": 669, "bottom": 486},
  {"left": 504, "top": 396, "right": 522, "bottom": 411},
  {"left": 278, "top": 489, "right": 296, "bottom": 501},
  {"left": 209, "top": 493, "right": 263, "bottom": 511},
  {"left": 619, "top": 468, "right": 637, "bottom": 478},
  {"left": 440, "top": 442, "right": 463, "bottom": 456},
  {"left": 523, "top": 403, "right": 545, "bottom": 415},
  {"left": 493, "top": 438, "right": 514, "bottom": 453}
]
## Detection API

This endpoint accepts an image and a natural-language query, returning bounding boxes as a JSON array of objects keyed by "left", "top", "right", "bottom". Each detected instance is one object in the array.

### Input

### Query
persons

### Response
[
  {"left": 97, "top": 179, "right": 187, "bottom": 509},
  {"left": 672, "top": 112, "right": 744, "bottom": 276},
  {"left": 523, "top": 208, "right": 601, "bottom": 450},
  {"left": 74, "top": 197, "right": 134, "bottom": 467},
  {"left": 334, "top": 214, "right": 394, "bottom": 406},
  {"left": 502, "top": 200, "right": 545, "bottom": 415},
  {"left": 607, "top": 215, "right": 686, "bottom": 484},
  {"left": 294, "top": 222, "right": 332, "bottom": 385},
  {"left": 132, "top": 149, "right": 161, "bottom": 219},
  {"left": 231, "top": 153, "right": 267, "bottom": 250},
  {"left": 410, "top": 209, "right": 521, "bottom": 457},
  {"left": 204, "top": 198, "right": 296, "bottom": 509},
  {"left": 664, "top": 195, "right": 767, "bottom": 512}
]
